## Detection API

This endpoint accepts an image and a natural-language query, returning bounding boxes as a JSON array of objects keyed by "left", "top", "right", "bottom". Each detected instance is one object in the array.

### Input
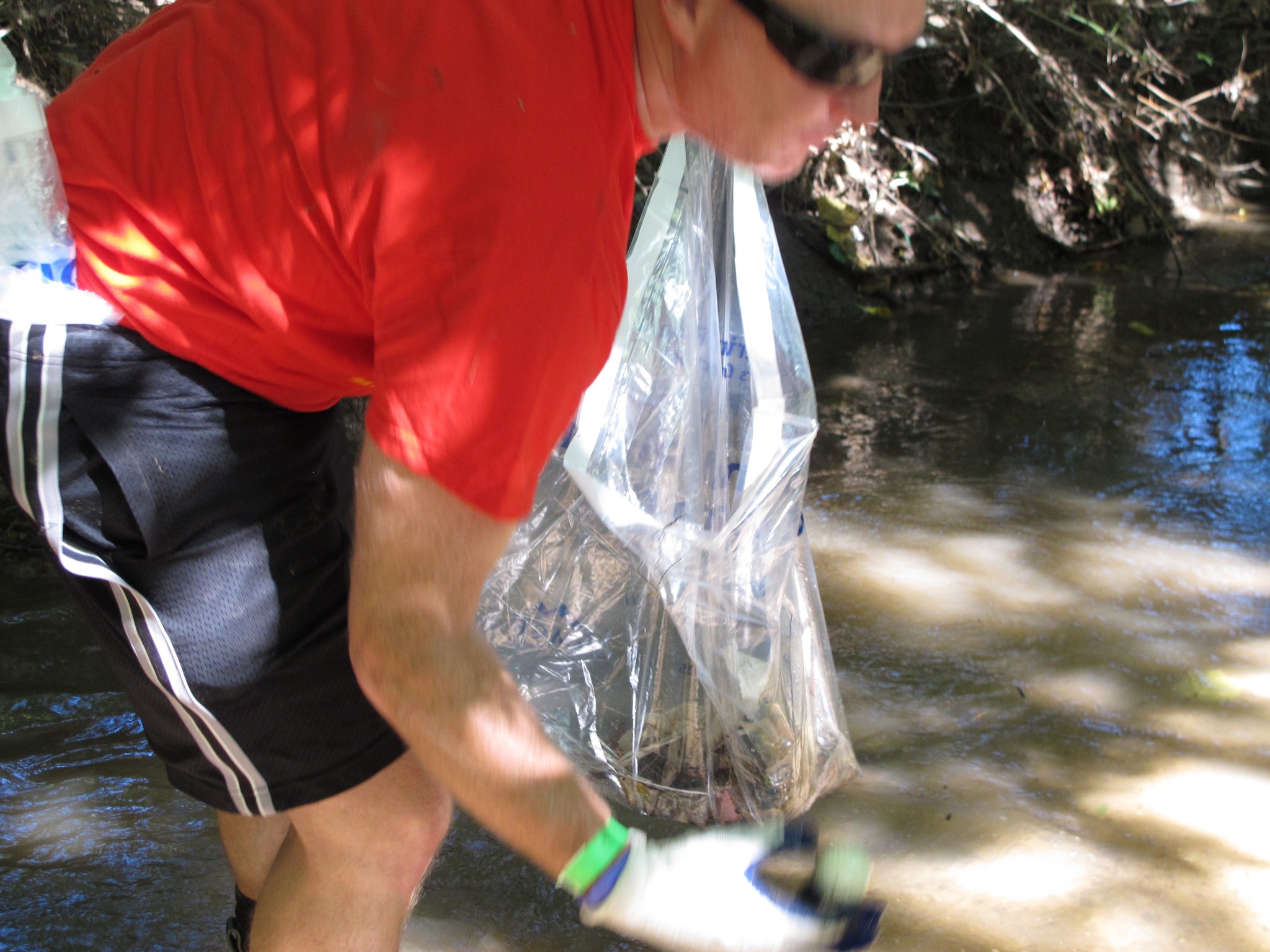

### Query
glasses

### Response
[{"left": 736, "top": 1, "right": 884, "bottom": 86}]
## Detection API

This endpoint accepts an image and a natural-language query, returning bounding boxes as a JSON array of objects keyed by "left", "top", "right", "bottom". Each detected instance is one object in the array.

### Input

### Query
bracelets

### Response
[{"left": 553, "top": 816, "right": 630, "bottom": 901}]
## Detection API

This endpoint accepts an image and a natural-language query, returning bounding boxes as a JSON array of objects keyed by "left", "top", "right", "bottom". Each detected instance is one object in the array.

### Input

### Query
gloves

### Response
[{"left": 579, "top": 809, "right": 884, "bottom": 952}]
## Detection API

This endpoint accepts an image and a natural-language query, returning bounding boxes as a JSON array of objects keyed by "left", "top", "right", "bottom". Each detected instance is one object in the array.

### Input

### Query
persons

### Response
[{"left": 0, "top": 0, "right": 929, "bottom": 952}]
